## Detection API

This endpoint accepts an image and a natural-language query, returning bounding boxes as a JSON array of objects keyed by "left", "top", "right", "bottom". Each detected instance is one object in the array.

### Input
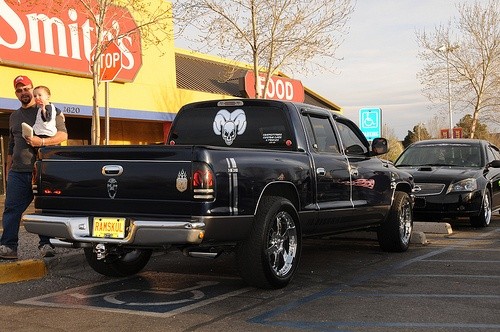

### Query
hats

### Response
[{"left": 14, "top": 76, "right": 32, "bottom": 89}]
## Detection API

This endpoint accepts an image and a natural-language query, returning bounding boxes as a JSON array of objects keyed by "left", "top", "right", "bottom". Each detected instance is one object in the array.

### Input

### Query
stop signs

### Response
[{"left": 89, "top": 40, "right": 121, "bottom": 82}]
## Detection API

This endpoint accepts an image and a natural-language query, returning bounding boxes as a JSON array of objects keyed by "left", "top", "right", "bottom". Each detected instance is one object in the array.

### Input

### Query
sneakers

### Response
[
  {"left": 0, "top": 244, "right": 18, "bottom": 259},
  {"left": 41, "top": 244, "right": 54, "bottom": 257}
]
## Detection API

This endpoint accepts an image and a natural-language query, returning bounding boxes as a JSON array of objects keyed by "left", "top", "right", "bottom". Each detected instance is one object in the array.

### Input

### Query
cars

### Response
[{"left": 393, "top": 138, "right": 500, "bottom": 229}]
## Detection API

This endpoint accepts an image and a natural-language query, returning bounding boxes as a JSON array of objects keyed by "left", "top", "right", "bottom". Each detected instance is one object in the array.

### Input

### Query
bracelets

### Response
[{"left": 42, "top": 138, "right": 44, "bottom": 147}]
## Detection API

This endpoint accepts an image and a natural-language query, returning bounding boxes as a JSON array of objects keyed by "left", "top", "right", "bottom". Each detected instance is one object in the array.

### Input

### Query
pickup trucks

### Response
[{"left": 20, "top": 97, "right": 416, "bottom": 290}]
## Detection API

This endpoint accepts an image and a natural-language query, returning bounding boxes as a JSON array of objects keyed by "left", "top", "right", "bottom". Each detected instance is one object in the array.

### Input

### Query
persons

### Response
[
  {"left": 22, "top": 86, "right": 65, "bottom": 139},
  {"left": 0, "top": 75, "right": 68, "bottom": 259}
]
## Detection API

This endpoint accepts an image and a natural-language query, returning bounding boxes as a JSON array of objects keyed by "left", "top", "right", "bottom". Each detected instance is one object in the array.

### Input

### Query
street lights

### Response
[{"left": 436, "top": 41, "right": 460, "bottom": 139}]
